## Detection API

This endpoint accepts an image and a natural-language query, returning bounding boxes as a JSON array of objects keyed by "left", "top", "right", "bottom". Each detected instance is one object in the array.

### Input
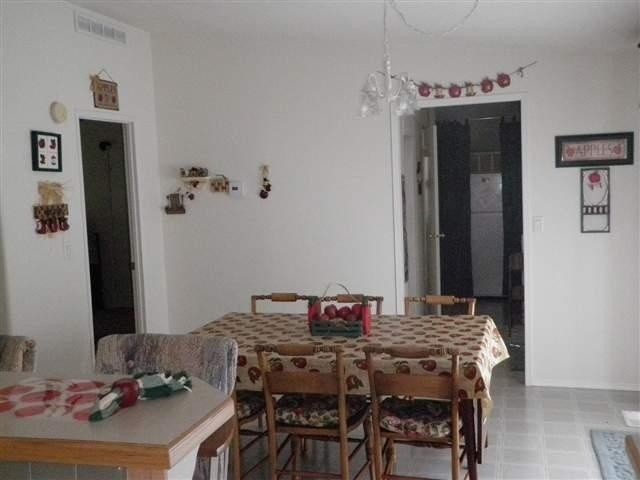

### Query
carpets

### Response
[{"left": 592, "top": 429, "right": 640, "bottom": 479}]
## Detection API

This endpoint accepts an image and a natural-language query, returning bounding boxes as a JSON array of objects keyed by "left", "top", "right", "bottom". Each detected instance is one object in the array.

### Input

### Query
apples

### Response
[
  {"left": 313, "top": 304, "right": 361, "bottom": 320},
  {"left": 0, "top": 381, "right": 104, "bottom": 421},
  {"left": 497, "top": 74, "right": 510, "bottom": 87},
  {"left": 481, "top": 80, "right": 492, "bottom": 92},
  {"left": 448, "top": 85, "right": 461, "bottom": 96},
  {"left": 113, "top": 378, "right": 139, "bottom": 406},
  {"left": 418, "top": 83, "right": 432, "bottom": 95}
]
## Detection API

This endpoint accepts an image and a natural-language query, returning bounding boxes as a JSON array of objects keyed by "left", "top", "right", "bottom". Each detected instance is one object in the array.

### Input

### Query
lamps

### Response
[{"left": 355, "top": 0, "right": 421, "bottom": 120}]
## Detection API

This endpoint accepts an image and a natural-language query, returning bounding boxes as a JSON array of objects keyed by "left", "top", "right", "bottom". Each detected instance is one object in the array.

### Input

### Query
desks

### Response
[
  {"left": 1, "top": 369, "right": 234, "bottom": 480},
  {"left": 187, "top": 312, "right": 489, "bottom": 463}
]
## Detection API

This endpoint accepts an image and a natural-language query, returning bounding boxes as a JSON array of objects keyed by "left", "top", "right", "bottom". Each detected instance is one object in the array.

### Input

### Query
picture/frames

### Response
[{"left": 555, "top": 132, "right": 633, "bottom": 167}]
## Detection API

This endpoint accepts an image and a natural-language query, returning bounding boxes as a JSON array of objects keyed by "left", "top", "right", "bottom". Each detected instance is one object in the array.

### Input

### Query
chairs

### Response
[
  {"left": 240, "top": 390, "right": 278, "bottom": 480},
  {"left": 250, "top": 295, "right": 320, "bottom": 315},
  {"left": 252, "top": 343, "right": 376, "bottom": 479},
  {"left": 94, "top": 334, "right": 237, "bottom": 480},
  {"left": 404, "top": 294, "right": 490, "bottom": 463},
  {"left": 318, "top": 294, "right": 384, "bottom": 313},
  {"left": 361, "top": 342, "right": 478, "bottom": 480}
]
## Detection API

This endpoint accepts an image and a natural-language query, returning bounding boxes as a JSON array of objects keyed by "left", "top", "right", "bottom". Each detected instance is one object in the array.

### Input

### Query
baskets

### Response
[{"left": 309, "top": 283, "right": 372, "bottom": 337}]
